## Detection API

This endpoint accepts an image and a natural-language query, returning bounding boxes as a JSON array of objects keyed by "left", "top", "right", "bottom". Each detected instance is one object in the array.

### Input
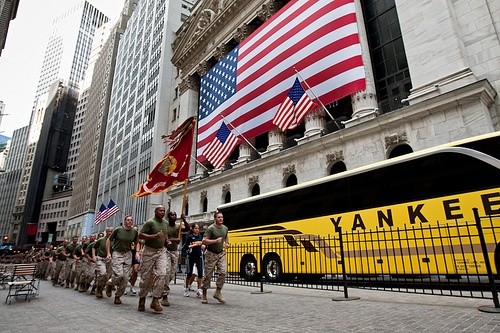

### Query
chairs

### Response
[{"left": 0, "top": 263, "right": 39, "bottom": 305}]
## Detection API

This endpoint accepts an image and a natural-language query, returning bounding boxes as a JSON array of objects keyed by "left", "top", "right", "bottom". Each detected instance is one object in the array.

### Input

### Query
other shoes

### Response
[
  {"left": 112, "top": 287, "right": 116, "bottom": 290},
  {"left": 35, "top": 273, "right": 91, "bottom": 292},
  {"left": 91, "top": 289, "right": 95, "bottom": 295},
  {"left": 189, "top": 287, "right": 194, "bottom": 290},
  {"left": 147, "top": 292, "right": 152, "bottom": 297},
  {"left": 129, "top": 278, "right": 132, "bottom": 283},
  {"left": 124, "top": 291, "right": 127, "bottom": 294},
  {"left": 130, "top": 287, "right": 136, "bottom": 294}
]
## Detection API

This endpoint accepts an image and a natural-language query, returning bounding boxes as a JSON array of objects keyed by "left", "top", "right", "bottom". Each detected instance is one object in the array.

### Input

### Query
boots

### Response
[
  {"left": 115, "top": 297, "right": 121, "bottom": 304},
  {"left": 138, "top": 297, "right": 145, "bottom": 310},
  {"left": 161, "top": 295, "right": 170, "bottom": 306},
  {"left": 213, "top": 288, "right": 226, "bottom": 303},
  {"left": 202, "top": 291, "right": 207, "bottom": 303},
  {"left": 106, "top": 286, "right": 112, "bottom": 296},
  {"left": 151, "top": 298, "right": 162, "bottom": 311},
  {"left": 96, "top": 286, "right": 103, "bottom": 298}
]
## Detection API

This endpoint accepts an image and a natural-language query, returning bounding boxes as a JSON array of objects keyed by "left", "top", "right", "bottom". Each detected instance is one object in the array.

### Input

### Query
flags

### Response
[
  {"left": 197, "top": 0, "right": 367, "bottom": 164},
  {"left": 130, "top": 115, "right": 195, "bottom": 197},
  {"left": 94, "top": 203, "right": 107, "bottom": 225},
  {"left": 272, "top": 76, "right": 317, "bottom": 133},
  {"left": 99, "top": 199, "right": 120, "bottom": 222},
  {"left": 202, "top": 121, "right": 244, "bottom": 169}
]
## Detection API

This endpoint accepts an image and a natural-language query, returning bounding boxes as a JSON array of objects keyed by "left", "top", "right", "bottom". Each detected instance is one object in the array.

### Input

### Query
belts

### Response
[{"left": 207, "top": 248, "right": 222, "bottom": 254}]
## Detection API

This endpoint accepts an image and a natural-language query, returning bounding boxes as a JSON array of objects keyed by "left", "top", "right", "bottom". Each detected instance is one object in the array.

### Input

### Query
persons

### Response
[
  {"left": 106, "top": 214, "right": 140, "bottom": 303},
  {"left": 161, "top": 211, "right": 190, "bottom": 306},
  {"left": 74, "top": 227, "right": 114, "bottom": 298},
  {"left": 185, "top": 211, "right": 230, "bottom": 304},
  {"left": 123, "top": 225, "right": 138, "bottom": 294},
  {"left": 0, "top": 236, "right": 79, "bottom": 288},
  {"left": 138, "top": 205, "right": 172, "bottom": 311}
]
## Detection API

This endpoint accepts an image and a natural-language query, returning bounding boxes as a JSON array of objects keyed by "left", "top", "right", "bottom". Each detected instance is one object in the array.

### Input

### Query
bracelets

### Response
[
  {"left": 183, "top": 219, "right": 186, "bottom": 223},
  {"left": 136, "top": 251, "right": 139, "bottom": 252}
]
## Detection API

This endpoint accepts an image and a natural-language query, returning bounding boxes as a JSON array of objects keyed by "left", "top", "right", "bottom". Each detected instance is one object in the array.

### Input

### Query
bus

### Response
[{"left": 215, "top": 130, "right": 500, "bottom": 282}]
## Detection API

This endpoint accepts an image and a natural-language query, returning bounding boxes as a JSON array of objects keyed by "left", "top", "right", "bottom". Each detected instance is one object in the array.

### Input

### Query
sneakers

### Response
[
  {"left": 184, "top": 288, "right": 189, "bottom": 296},
  {"left": 197, "top": 289, "right": 203, "bottom": 298}
]
